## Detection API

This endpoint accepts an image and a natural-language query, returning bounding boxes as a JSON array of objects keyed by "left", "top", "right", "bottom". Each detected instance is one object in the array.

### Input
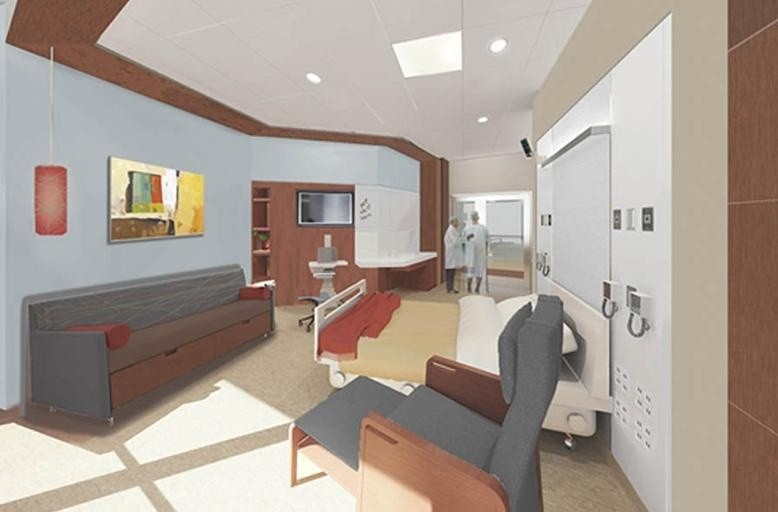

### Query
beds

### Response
[{"left": 314, "top": 280, "right": 612, "bottom": 450}]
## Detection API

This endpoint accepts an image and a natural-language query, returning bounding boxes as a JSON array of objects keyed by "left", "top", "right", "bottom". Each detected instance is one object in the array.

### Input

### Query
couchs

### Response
[{"left": 24, "top": 265, "right": 274, "bottom": 429}]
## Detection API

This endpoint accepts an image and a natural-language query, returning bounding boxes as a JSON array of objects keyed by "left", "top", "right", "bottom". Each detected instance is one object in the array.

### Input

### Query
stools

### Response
[{"left": 290, "top": 376, "right": 408, "bottom": 500}]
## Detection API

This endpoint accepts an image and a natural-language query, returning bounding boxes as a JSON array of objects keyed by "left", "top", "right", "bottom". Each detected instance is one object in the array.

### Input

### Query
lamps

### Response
[{"left": 35, "top": 45, "right": 68, "bottom": 235}]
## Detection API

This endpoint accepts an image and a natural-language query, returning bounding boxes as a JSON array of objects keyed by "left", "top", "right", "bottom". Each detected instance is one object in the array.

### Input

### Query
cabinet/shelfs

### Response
[{"left": 251, "top": 184, "right": 272, "bottom": 281}]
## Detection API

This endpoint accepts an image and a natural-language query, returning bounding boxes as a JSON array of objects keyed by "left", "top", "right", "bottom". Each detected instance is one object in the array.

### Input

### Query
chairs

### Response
[{"left": 357, "top": 294, "right": 561, "bottom": 512}]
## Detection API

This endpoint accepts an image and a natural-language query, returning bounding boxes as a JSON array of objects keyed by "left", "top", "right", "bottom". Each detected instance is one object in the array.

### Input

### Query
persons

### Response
[
  {"left": 460, "top": 211, "right": 491, "bottom": 294},
  {"left": 443, "top": 215, "right": 475, "bottom": 294}
]
